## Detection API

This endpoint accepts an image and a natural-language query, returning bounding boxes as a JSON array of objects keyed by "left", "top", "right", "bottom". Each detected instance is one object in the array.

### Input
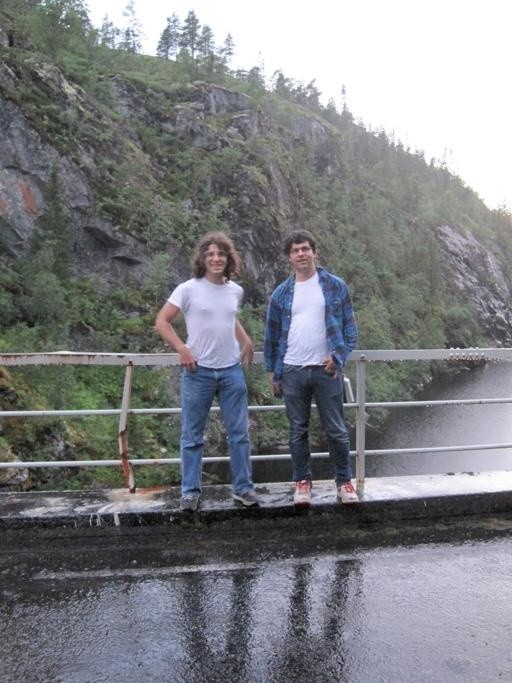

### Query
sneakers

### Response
[
  {"left": 293, "top": 479, "right": 313, "bottom": 507},
  {"left": 335, "top": 480, "right": 360, "bottom": 505},
  {"left": 231, "top": 489, "right": 260, "bottom": 507},
  {"left": 179, "top": 495, "right": 199, "bottom": 512}
]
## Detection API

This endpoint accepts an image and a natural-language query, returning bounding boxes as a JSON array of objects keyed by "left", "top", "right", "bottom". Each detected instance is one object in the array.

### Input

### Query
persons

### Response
[
  {"left": 263, "top": 231, "right": 360, "bottom": 506},
  {"left": 155, "top": 231, "right": 264, "bottom": 511}
]
